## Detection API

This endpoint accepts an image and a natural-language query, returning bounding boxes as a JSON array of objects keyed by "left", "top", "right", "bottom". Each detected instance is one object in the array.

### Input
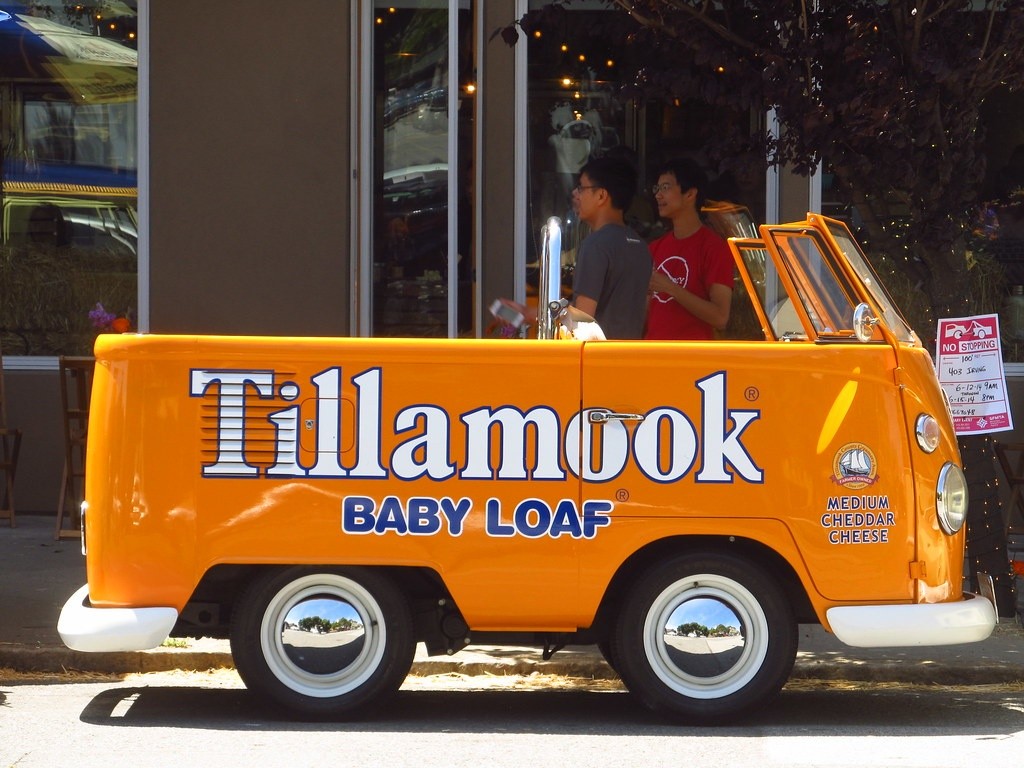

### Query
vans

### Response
[{"left": 57, "top": 212, "right": 999, "bottom": 726}]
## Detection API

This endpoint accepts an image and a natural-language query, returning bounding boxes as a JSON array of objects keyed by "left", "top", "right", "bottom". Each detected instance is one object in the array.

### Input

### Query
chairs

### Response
[
  {"left": 54, "top": 355, "right": 97, "bottom": 540},
  {"left": 0, "top": 347, "right": 22, "bottom": 529}
]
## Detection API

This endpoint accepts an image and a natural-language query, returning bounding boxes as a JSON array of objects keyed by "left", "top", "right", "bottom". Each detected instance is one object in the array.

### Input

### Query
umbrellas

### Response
[{"left": 0, "top": 9, "right": 138, "bottom": 104}]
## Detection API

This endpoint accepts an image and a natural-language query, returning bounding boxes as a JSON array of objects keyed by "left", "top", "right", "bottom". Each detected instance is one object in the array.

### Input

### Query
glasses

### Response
[{"left": 577, "top": 185, "right": 597, "bottom": 193}]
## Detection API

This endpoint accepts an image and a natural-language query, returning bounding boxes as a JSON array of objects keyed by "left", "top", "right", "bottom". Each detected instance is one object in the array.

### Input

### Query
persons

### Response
[
  {"left": 735, "top": 144, "right": 766, "bottom": 217},
  {"left": 640, "top": 157, "right": 736, "bottom": 340},
  {"left": 458, "top": 160, "right": 472, "bottom": 332},
  {"left": 562, "top": 158, "right": 653, "bottom": 339}
]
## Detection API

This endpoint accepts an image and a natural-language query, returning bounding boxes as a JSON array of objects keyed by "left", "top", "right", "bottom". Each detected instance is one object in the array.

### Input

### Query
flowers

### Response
[{"left": 87, "top": 302, "right": 131, "bottom": 333}]
